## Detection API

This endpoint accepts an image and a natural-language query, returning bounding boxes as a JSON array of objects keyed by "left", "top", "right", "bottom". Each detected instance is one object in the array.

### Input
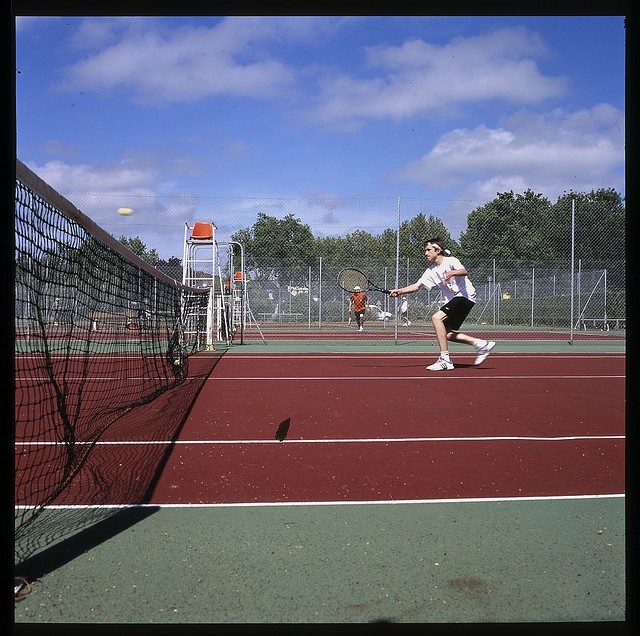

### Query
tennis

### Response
[
  {"left": 174, "top": 359, "right": 181, "bottom": 366},
  {"left": 118, "top": 207, "right": 132, "bottom": 215}
]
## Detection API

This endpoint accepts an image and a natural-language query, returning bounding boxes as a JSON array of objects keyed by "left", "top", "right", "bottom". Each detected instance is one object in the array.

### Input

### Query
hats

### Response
[{"left": 353, "top": 285, "right": 360, "bottom": 290}]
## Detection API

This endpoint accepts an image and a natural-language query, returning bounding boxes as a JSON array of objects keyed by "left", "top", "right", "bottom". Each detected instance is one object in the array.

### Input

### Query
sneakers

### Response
[
  {"left": 426, "top": 357, "right": 455, "bottom": 372},
  {"left": 474, "top": 340, "right": 496, "bottom": 365}
]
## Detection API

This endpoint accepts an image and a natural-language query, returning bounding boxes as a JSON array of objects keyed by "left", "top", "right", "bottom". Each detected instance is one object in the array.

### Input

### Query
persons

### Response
[
  {"left": 349, "top": 285, "right": 369, "bottom": 331},
  {"left": 388, "top": 237, "right": 496, "bottom": 371},
  {"left": 400, "top": 297, "right": 411, "bottom": 326}
]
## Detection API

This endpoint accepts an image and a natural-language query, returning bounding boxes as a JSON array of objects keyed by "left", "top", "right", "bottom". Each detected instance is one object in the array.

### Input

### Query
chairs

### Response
[
  {"left": 184, "top": 220, "right": 218, "bottom": 244},
  {"left": 233, "top": 271, "right": 244, "bottom": 280}
]
